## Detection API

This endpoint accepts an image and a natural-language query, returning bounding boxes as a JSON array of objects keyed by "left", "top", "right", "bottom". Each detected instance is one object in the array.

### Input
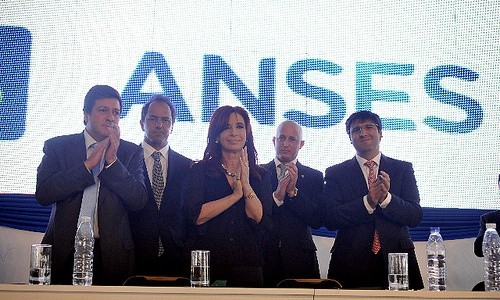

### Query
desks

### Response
[
  {"left": 314, "top": 288, "right": 500, "bottom": 300},
  {"left": 0, "top": 283, "right": 315, "bottom": 300}
]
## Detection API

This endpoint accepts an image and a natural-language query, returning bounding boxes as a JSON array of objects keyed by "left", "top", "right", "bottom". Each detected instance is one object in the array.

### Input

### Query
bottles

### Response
[
  {"left": 426, "top": 227, "right": 447, "bottom": 292},
  {"left": 72, "top": 216, "right": 95, "bottom": 286},
  {"left": 483, "top": 223, "right": 500, "bottom": 291}
]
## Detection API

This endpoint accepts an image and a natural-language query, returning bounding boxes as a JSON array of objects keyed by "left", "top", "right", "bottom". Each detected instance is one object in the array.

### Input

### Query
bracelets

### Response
[{"left": 244, "top": 191, "right": 255, "bottom": 199}]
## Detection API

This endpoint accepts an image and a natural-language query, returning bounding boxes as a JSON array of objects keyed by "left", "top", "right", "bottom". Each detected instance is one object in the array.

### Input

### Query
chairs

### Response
[
  {"left": 123, "top": 275, "right": 190, "bottom": 286},
  {"left": 278, "top": 277, "right": 343, "bottom": 289}
]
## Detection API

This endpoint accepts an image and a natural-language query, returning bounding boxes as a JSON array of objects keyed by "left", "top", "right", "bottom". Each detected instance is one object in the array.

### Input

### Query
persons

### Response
[
  {"left": 35, "top": 85, "right": 148, "bottom": 286},
  {"left": 257, "top": 120, "right": 323, "bottom": 288},
  {"left": 128, "top": 93, "right": 196, "bottom": 286},
  {"left": 474, "top": 173, "right": 500, "bottom": 258},
  {"left": 182, "top": 106, "right": 273, "bottom": 287},
  {"left": 323, "top": 111, "right": 425, "bottom": 290}
]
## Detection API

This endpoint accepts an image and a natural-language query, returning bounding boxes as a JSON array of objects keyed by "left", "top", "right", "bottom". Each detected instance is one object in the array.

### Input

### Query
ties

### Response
[
  {"left": 151, "top": 152, "right": 164, "bottom": 256},
  {"left": 277, "top": 164, "right": 288, "bottom": 186},
  {"left": 364, "top": 161, "right": 382, "bottom": 254},
  {"left": 77, "top": 143, "right": 100, "bottom": 233}
]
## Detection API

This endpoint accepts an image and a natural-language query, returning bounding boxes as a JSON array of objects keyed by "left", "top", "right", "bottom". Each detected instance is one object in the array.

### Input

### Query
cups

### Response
[
  {"left": 388, "top": 253, "right": 408, "bottom": 292},
  {"left": 191, "top": 250, "right": 210, "bottom": 288},
  {"left": 29, "top": 243, "right": 51, "bottom": 285}
]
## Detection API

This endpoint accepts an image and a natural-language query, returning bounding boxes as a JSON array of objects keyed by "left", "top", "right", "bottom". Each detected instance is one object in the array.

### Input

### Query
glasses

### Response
[{"left": 347, "top": 124, "right": 379, "bottom": 135}]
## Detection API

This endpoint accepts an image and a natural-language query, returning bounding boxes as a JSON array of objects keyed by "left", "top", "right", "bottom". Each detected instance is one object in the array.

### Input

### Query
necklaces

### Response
[{"left": 221, "top": 162, "right": 236, "bottom": 177}]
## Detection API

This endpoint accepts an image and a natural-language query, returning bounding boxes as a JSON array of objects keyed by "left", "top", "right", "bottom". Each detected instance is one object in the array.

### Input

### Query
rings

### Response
[
  {"left": 245, "top": 162, "right": 247, "bottom": 167},
  {"left": 236, "top": 176, "right": 240, "bottom": 180}
]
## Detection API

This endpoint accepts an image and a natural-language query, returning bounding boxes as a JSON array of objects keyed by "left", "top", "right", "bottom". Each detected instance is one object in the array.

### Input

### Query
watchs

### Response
[{"left": 288, "top": 186, "right": 297, "bottom": 199}]
